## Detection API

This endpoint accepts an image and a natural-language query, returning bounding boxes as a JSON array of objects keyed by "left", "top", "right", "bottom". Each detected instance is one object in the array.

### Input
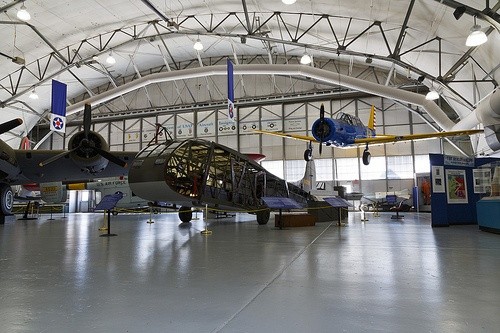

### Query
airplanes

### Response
[
  {"left": 124, "top": 122, "right": 364, "bottom": 226},
  {"left": 0, "top": 102, "right": 266, "bottom": 217},
  {"left": 244, "top": 103, "right": 485, "bottom": 166}
]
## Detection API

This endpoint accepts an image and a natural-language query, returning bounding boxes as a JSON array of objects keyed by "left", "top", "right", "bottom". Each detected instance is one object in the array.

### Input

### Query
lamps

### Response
[
  {"left": 194, "top": 34, "right": 203, "bottom": 50},
  {"left": 466, "top": 16, "right": 487, "bottom": 46},
  {"left": 106, "top": 50, "right": 115, "bottom": 63},
  {"left": 17, "top": 2, "right": 31, "bottom": 20},
  {"left": 300, "top": 46, "right": 311, "bottom": 64},
  {"left": 425, "top": 79, "right": 439, "bottom": 100}
]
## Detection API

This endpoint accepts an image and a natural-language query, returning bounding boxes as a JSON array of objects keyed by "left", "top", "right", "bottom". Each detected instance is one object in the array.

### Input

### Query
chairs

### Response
[{"left": 390, "top": 199, "right": 405, "bottom": 218}]
